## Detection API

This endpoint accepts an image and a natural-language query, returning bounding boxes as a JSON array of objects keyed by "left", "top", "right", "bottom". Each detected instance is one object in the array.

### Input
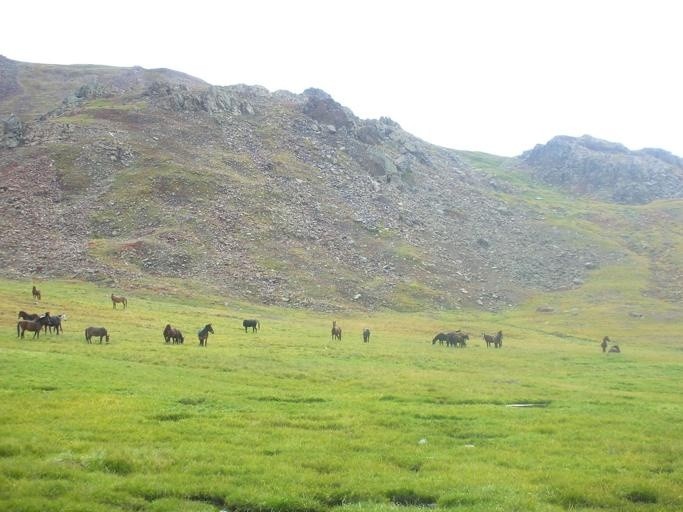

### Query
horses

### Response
[
  {"left": 17, "top": 311, "right": 64, "bottom": 340},
  {"left": 85, "top": 327, "right": 109, "bottom": 344},
  {"left": 32, "top": 286, "right": 41, "bottom": 299},
  {"left": 163, "top": 324, "right": 183, "bottom": 344},
  {"left": 111, "top": 294, "right": 127, "bottom": 309},
  {"left": 332, "top": 320, "right": 341, "bottom": 341},
  {"left": 198, "top": 323, "right": 214, "bottom": 347},
  {"left": 601, "top": 336, "right": 621, "bottom": 353},
  {"left": 243, "top": 320, "right": 260, "bottom": 333},
  {"left": 432, "top": 330, "right": 503, "bottom": 349},
  {"left": 363, "top": 328, "right": 370, "bottom": 342}
]
